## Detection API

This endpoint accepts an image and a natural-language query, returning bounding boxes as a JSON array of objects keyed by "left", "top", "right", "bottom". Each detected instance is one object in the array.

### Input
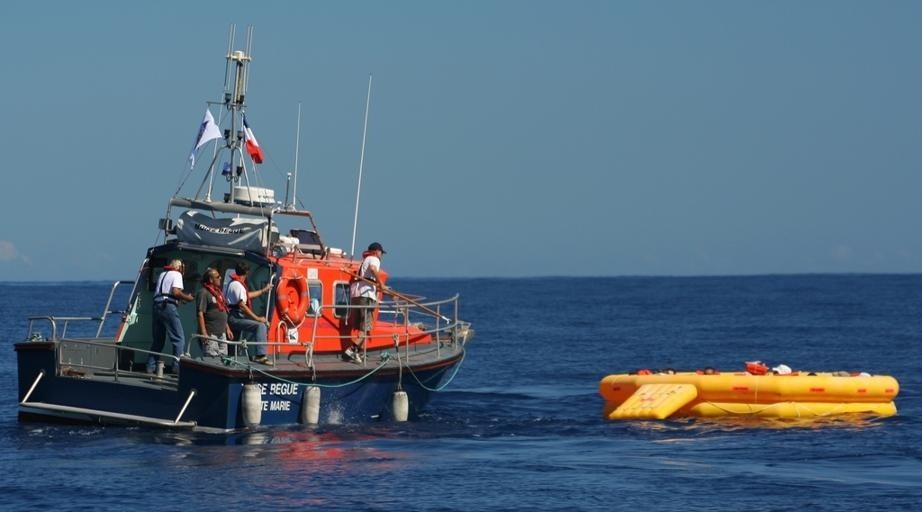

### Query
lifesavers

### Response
[{"left": 276, "top": 271, "right": 310, "bottom": 327}]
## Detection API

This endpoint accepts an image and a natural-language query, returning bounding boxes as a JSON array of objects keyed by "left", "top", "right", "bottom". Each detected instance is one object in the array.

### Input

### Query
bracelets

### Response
[{"left": 261, "top": 288, "right": 265, "bottom": 295}]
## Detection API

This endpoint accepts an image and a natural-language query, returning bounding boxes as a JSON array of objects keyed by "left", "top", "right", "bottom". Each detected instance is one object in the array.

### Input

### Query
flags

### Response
[
  {"left": 188, "top": 107, "right": 223, "bottom": 171},
  {"left": 241, "top": 114, "right": 264, "bottom": 164}
]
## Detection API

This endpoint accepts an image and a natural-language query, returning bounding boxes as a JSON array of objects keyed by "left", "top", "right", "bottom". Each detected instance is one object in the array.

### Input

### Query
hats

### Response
[
  {"left": 772, "top": 364, "right": 792, "bottom": 375},
  {"left": 368, "top": 242, "right": 386, "bottom": 253}
]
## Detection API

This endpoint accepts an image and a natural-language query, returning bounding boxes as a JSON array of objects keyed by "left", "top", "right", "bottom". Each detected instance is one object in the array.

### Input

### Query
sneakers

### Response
[
  {"left": 254, "top": 355, "right": 274, "bottom": 366},
  {"left": 345, "top": 346, "right": 362, "bottom": 364}
]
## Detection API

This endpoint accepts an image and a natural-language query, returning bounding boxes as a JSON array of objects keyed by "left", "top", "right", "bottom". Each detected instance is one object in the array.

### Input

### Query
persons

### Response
[
  {"left": 144, "top": 257, "right": 195, "bottom": 375},
  {"left": 343, "top": 241, "right": 393, "bottom": 365},
  {"left": 223, "top": 260, "right": 276, "bottom": 365},
  {"left": 627, "top": 359, "right": 871, "bottom": 382},
  {"left": 196, "top": 266, "right": 235, "bottom": 356}
]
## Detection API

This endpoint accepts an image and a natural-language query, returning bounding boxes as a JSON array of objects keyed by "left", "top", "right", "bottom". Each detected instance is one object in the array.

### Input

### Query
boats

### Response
[
  {"left": 14, "top": 20, "right": 475, "bottom": 434},
  {"left": 592, "top": 361, "right": 898, "bottom": 420}
]
